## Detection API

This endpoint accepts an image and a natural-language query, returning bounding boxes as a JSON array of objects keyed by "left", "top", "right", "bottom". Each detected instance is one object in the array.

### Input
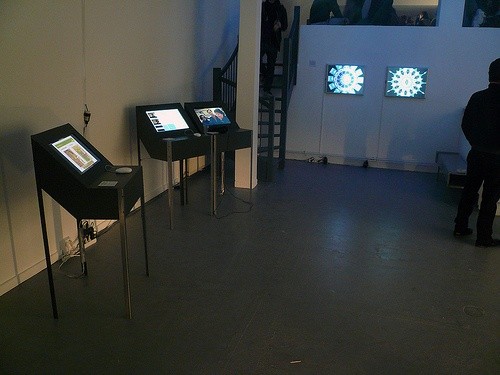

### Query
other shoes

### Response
[
  {"left": 475, "top": 237, "right": 500, "bottom": 247},
  {"left": 453, "top": 222, "right": 473, "bottom": 239}
]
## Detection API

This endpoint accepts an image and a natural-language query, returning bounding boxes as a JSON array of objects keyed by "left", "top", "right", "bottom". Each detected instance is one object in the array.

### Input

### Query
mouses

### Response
[
  {"left": 194, "top": 133, "right": 201, "bottom": 137},
  {"left": 116, "top": 167, "right": 132, "bottom": 173}
]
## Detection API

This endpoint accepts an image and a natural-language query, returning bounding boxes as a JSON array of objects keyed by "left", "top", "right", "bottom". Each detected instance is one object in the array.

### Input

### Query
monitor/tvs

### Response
[
  {"left": 49, "top": 133, "right": 101, "bottom": 176},
  {"left": 194, "top": 107, "right": 232, "bottom": 126},
  {"left": 146, "top": 108, "right": 190, "bottom": 134}
]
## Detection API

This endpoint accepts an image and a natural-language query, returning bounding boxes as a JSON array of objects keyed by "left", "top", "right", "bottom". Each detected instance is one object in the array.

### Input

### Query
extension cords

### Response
[{"left": 83, "top": 238, "right": 97, "bottom": 248}]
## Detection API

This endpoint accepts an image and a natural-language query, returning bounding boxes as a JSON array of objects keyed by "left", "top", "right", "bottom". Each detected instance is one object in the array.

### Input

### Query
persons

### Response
[
  {"left": 453, "top": 59, "right": 499, "bottom": 247},
  {"left": 200, "top": 109, "right": 230, "bottom": 127}
]
